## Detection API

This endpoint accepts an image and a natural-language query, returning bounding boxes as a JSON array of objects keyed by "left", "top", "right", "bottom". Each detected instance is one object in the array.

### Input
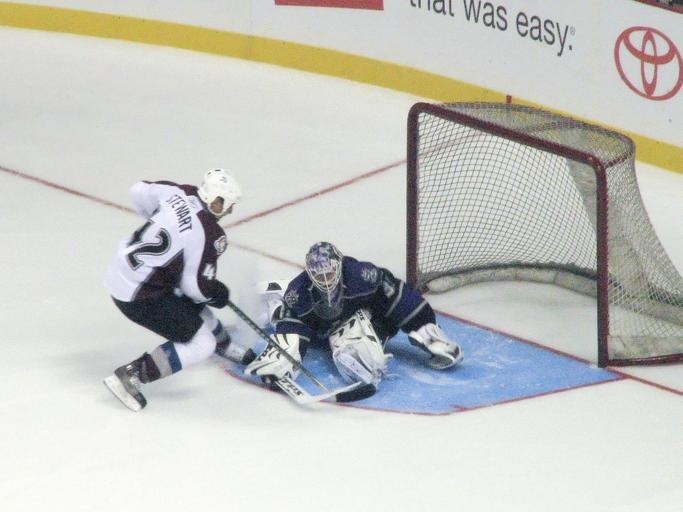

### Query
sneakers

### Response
[
  {"left": 214, "top": 332, "right": 255, "bottom": 365},
  {"left": 113, "top": 360, "right": 147, "bottom": 407}
]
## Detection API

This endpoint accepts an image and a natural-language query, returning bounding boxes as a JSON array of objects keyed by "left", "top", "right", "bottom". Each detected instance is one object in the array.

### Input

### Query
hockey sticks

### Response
[
  {"left": 273, "top": 375, "right": 363, "bottom": 406},
  {"left": 227, "top": 300, "right": 376, "bottom": 402}
]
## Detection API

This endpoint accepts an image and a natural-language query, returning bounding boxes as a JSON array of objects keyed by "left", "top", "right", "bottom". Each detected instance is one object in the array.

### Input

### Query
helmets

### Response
[
  {"left": 303, "top": 241, "right": 342, "bottom": 294},
  {"left": 196, "top": 167, "right": 243, "bottom": 221}
]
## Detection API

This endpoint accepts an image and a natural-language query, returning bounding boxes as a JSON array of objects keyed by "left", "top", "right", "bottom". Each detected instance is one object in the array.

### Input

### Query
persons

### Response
[
  {"left": 243, "top": 242, "right": 463, "bottom": 392},
  {"left": 103, "top": 168, "right": 257, "bottom": 412}
]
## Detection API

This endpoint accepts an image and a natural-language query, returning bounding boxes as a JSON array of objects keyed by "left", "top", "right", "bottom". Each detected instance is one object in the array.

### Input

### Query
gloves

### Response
[{"left": 207, "top": 277, "right": 229, "bottom": 309}]
